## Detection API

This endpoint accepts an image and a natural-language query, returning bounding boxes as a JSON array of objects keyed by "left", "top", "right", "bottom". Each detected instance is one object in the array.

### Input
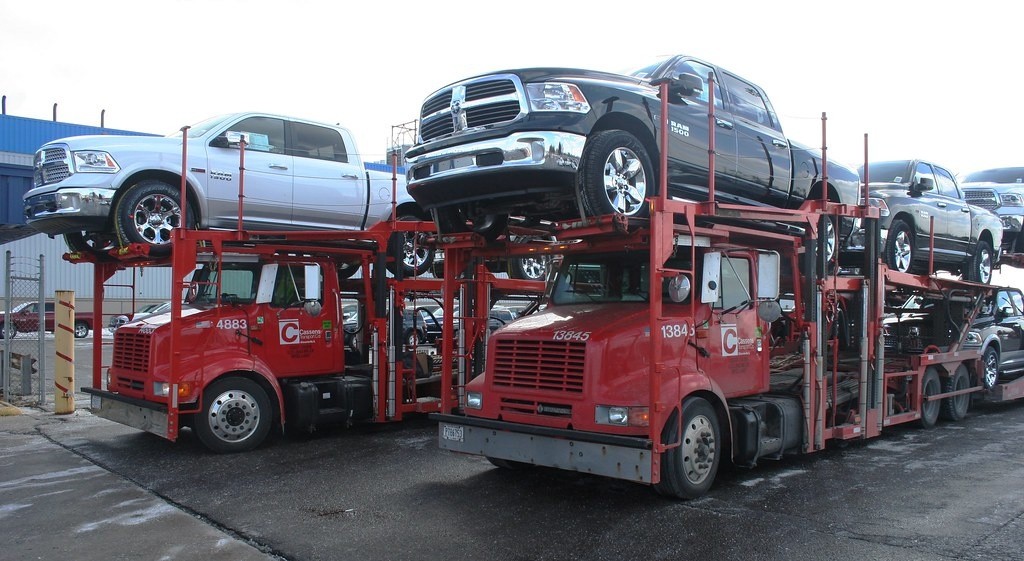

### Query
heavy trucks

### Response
[
  {"left": 432, "top": 83, "right": 1023, "bottom": 500},
  {"left": 64, "top": 124, "right": 554, "bottom": 454}
]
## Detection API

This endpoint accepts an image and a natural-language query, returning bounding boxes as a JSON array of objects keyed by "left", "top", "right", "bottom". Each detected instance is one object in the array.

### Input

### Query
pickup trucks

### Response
[
  {"left": 402, "top": 55, "right": 862, "bottom": 248},
  {"left": 834, "top": 161, "right": 1004, "bottom": 282},
  {"left": 0, "top": 301, "right": 94, "bottom": 340},
  {"left": 22, "top": 112, "right": 415, "bottom": 257}
]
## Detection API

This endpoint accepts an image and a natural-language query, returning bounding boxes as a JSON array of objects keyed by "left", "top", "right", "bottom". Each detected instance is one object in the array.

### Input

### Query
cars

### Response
[
  {"left": 111, "top": 301, "right": 171, "bottom": 332},
  {"left": 329, "top": 300, "right": 532, "bottom": 364},
  {"left": 881, "top": 284, "right": 1024, "bottom": 385},
  {"left": 964, "top": 167, "right": 1024, "bottom": 257}
]
